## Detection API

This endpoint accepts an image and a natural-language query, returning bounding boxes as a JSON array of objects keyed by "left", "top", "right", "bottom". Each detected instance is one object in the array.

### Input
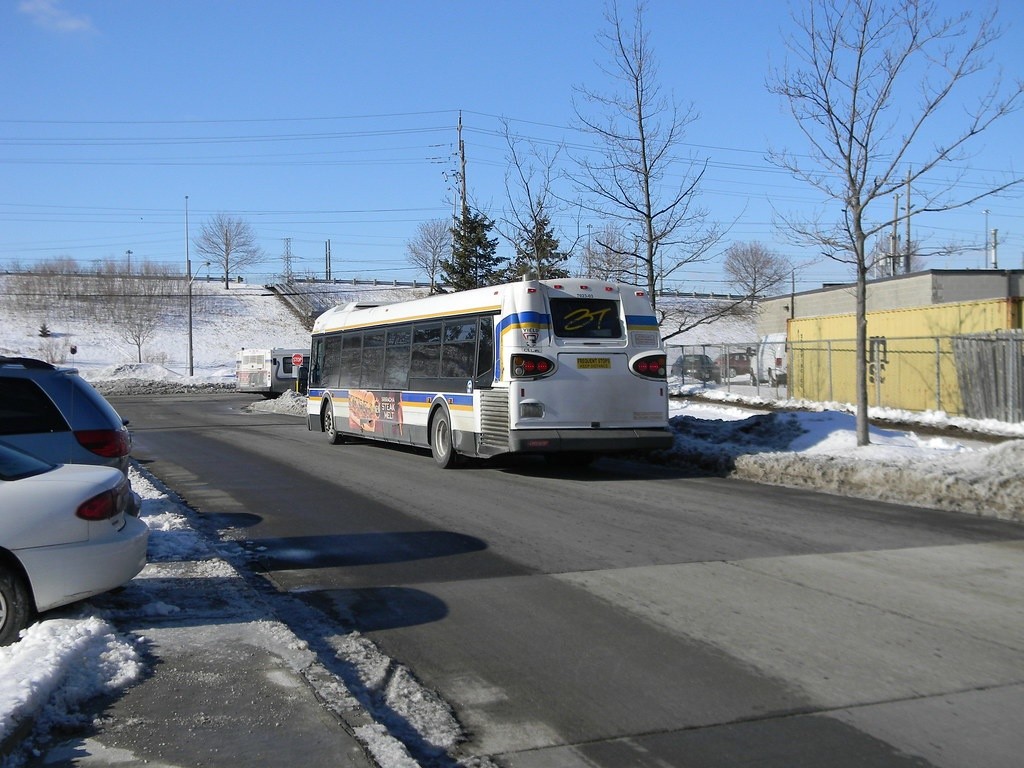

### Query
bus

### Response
[
  {"left": 235, "top": 347, "right": 311, "bottom": 398},
  {"left": 303, "top": 273, "right": 676, "bottom": 468}
]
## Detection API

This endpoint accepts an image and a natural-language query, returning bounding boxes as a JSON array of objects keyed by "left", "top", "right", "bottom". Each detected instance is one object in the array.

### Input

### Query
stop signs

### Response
[{"left": 292, "top": 354, "right": 303, "bottom": 367}]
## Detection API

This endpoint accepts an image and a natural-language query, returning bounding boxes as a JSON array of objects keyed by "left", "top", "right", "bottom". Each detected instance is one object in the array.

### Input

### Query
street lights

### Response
[
  {"left": 982, "top": 207, "right": 991, "bottom": 268},
  {"left": 873, "top": 234, "right": 877, "bottom": 280},
  {"left": 184, "top": 193, "right": 211, "bottom": 376},
  {"left": 125, "top": 249, "right": 134, "bottom": 275}
]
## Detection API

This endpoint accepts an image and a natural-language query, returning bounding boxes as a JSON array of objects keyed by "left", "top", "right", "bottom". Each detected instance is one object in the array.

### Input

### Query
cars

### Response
[
  {"left": 748, "top": 333, "right": 788, "bottom": 387},
  {"left": 714, "top": 352, "right": 749, "bottom": 378},
  {"left": 0, "top": 354, "right": 148, "bottom": 645},
  {"left": 671, "top": 354, "right": 721, "bottom": 385}
]
